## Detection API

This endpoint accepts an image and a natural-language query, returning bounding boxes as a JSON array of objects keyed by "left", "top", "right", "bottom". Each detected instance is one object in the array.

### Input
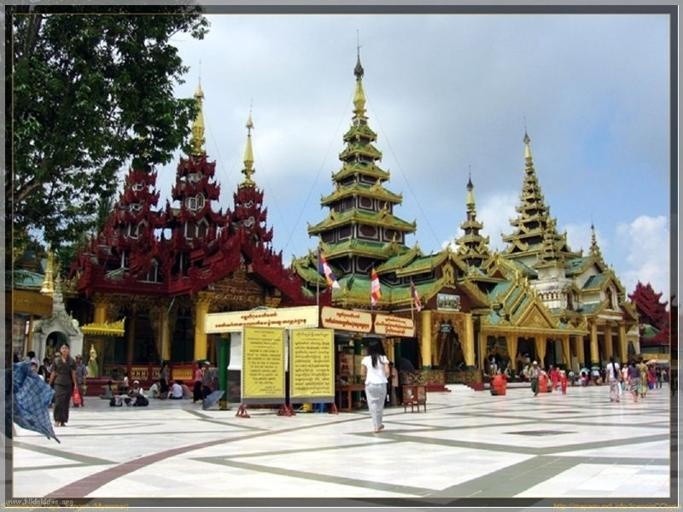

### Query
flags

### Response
[
  {"left": 317, "top": 250, "right": 340, "bottom": 289},
  {"left": 371, "top": 267, "right": 382, "bottom": 305},
  {"left": 410, "top": 281, "right": 421, "bottom": 311}
]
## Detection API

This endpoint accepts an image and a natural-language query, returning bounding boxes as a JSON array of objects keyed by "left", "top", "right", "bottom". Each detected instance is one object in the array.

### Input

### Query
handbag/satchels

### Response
[
  {"left": 73, "top": 387, "right": 82, "bottom": 404},
  {"left": 614, "top": 370, "right": 624, "bottom": 383}
]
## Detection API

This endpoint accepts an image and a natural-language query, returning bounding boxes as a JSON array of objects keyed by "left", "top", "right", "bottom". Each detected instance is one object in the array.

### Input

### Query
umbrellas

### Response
[
  {"left": 12, "top": 377, "right": 61, "bottom": 443},
  {"left": 12, "top": 360, "right": 50, "bottom": 440}
]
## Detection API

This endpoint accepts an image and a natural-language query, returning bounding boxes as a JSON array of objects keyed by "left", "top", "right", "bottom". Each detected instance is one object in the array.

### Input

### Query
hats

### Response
[
  {"left": 532, "top": 360, "right": 538, "bottom": 365},
  {"left": 204, "top": 361, "right": 210, "bottom": 366},
  {"left": 132, "top": 380, "right": 140, "bottom": 384}
]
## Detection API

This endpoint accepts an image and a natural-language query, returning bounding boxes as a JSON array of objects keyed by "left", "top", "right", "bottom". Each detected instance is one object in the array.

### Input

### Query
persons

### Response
[
  {"left": 14, "top": 342, "right": 219, "bottom": 407},
  {"left": 47, "top": 343, "right": 78, "bottom": 426},
  {"left": 399, "top": 353, "right": 415, "bottom": 406},
  {"left": 389, "top": 360, "right": 399, "bottom": 408},
  {"left": 360, "top": 339, "right": 390, "bottom": 433},
  {"left": 336, "top": 373, "right": 349, "bottom": 408},
  {"left": 487, "top": 349, "right": 668, "bottom": 403}
]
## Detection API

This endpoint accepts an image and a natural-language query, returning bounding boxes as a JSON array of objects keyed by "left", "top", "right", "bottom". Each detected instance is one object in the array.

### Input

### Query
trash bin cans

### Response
[
  {"left": 491, "top": 374, "right": 505, "bottom": 394},
  {"left": 403, "top": 386, "right": 426, "bottom": 413},
  {"left": 538, "top": 375, "right": 547, "bottom": 392}
]
